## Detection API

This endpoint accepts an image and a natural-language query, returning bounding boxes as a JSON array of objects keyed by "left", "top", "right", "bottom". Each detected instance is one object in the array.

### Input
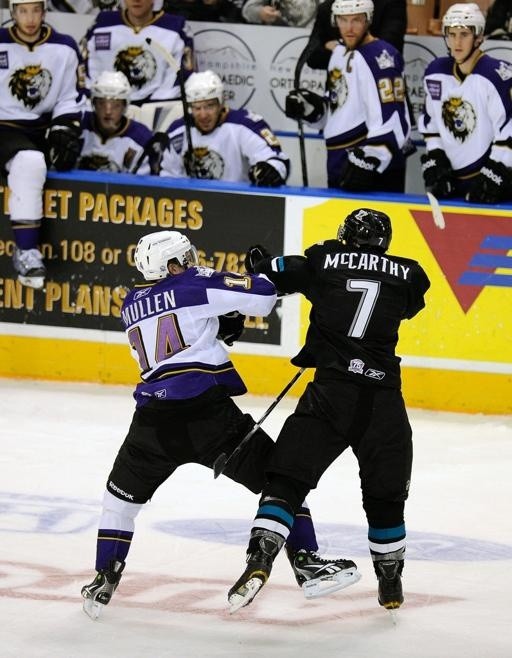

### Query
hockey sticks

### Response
[{"left": 213, "top": 367, "right": 309, "bottom": 479}]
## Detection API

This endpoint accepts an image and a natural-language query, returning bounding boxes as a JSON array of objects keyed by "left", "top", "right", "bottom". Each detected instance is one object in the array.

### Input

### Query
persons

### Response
[
  {"left": 1, "top": 1, "right": 85, "bottom": 290},
  {"left": 78, "top": 71, "right": 158, "bottom": 176},
  {"left": 483, "top": 0, "right": 512, "bottom": 41},
  {"left": 293, "top": 0, "right": 407, "bottom": 88},
  {"left": 286, "top": 0, "right": 409, "bottom": 196},
  {"left": 418, "top": 2, "right": 511, "bottom": 203},
  {"left": 79, "top": 225, "right": 363, "bottom": 607},
  {"left": 162, "top": 70, "right": 292, "bottom": 188},
  {"left": 78, "top": 1, "right": 197, "bottom": 102},
  {"left": 222, "top": 206, "right": 433, "bottom": 611},
  {"left": 1, "top": 1, "right": 318, "bottom": 29}
]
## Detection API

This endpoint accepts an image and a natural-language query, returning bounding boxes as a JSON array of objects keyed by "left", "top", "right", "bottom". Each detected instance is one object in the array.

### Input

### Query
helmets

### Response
[
  {"left": 10, "top": 0, "right": 47, "bottom": 12},
  {"left": 133, "top": 231, "right": 198, "bottom": 281},
  {"left": 338, "top": 208, "right": 392, "bottom": 248},
  {"left": 442, "top": 3, "right": 485, "bottom": 39},
  {"left": 185, "top": 69, "right": 224, "bottom": 104},
  {"left": 92, "top": 71, "right": 131, "bottom": 104},
  {"left": 331, "top": 0, "right": 374, "bottom": 29}
]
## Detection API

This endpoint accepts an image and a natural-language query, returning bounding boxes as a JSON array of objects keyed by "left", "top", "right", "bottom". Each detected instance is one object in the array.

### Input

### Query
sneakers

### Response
[
  {"left": 82, "top": 571, "right": 119, "bottom": 604},
  {"left": 228, "top": 560, "right": 270, "bottom": 606},
  {"left": 293, "top": 549, "right": 357, "bottom": 586},
  {"left": 14, "top": 249, "right": 47, "bottom": 276},
  {"left": 378, "top": 560, "right": 404, "bottom": 608}
]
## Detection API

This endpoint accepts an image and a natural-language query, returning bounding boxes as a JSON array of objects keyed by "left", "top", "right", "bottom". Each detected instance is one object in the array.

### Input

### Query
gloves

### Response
[
  {"left": 248, "top": 161, "right": 284, "bottom": 186},
  {"left": 216, "top": 313, "right": 246, "bottom": 346},
  {"left": 286, "top": 88, "right": 324, "bottom": 122},
  {"left": 338, "top": 147, "right": 509, "bottom": 204}
]
